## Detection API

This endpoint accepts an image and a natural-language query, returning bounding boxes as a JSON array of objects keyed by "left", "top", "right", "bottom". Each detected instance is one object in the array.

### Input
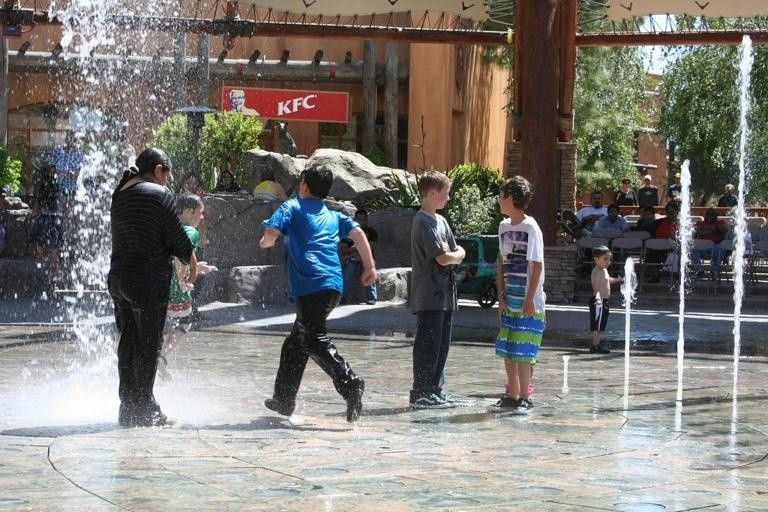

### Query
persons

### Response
[
  {"left": 174, "top": 172, "right": 212, "bottom": 323},
  {"left": 157, "top": 192, "right": 218, "bottom": 380},
  {"left": 25, "top": 125, "right": 138, "bottom": 294},
  {"left": 576, "top": 172, "right": 755, "bottom": 284},
  {"left": 339, "top": 211, "right": 378, "bottom": 307},
  {"left": 486, "top": 174, "right": 548, "bottom": 416},
  {"left": 406, "top": 169, "right": 466, "bottom": 408},
  {"left": 210, "top": 170, "right": 242, "bottom": 193},
  {"left": 229, "top": 90, "right": 261, "bottom": 118},
  {"left": 252, "top": 168, "right": 286, "bottom": 199},
  {"left": 108, "top": 146, "right": 198, "bottom": 429},
  {"left": 588, "top": 246, "right": 625, "bottom": 352},
  {"left": 259, "top": 164, "right": 382, "bottom": 424}
]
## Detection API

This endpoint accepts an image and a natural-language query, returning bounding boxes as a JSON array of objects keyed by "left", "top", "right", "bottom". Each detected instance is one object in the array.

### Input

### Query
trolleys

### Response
[{"left": 445, "top": 234, "right": 500, "bottom": 308}]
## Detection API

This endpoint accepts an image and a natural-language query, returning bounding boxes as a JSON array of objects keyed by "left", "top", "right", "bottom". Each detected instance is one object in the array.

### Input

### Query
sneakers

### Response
[
  {"left": 342, "top": 377, "right": 363, "bottom": 422},
  {"left": 515, "top": 399, "right": 534, "bottom": 414},
  {"left": 590, "top": 343, "right": 609, "bottom": 353},
  {"left": 265, "top": 399, "right": 295, "bottom": 416},
  {"left": 487, "top": 398, "right": 514, "bottom": 411},
  {"left": 410, "top": 390, "right": 450, "bottom": 409}
]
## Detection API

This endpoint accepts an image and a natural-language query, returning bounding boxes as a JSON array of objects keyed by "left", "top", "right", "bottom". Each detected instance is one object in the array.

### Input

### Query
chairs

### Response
[
  {"left": 254, "top": 192, "right": 280, "bottom": 202},
  {"left": 574, "top": 212, "right": 767, "bottom": 306}
]
